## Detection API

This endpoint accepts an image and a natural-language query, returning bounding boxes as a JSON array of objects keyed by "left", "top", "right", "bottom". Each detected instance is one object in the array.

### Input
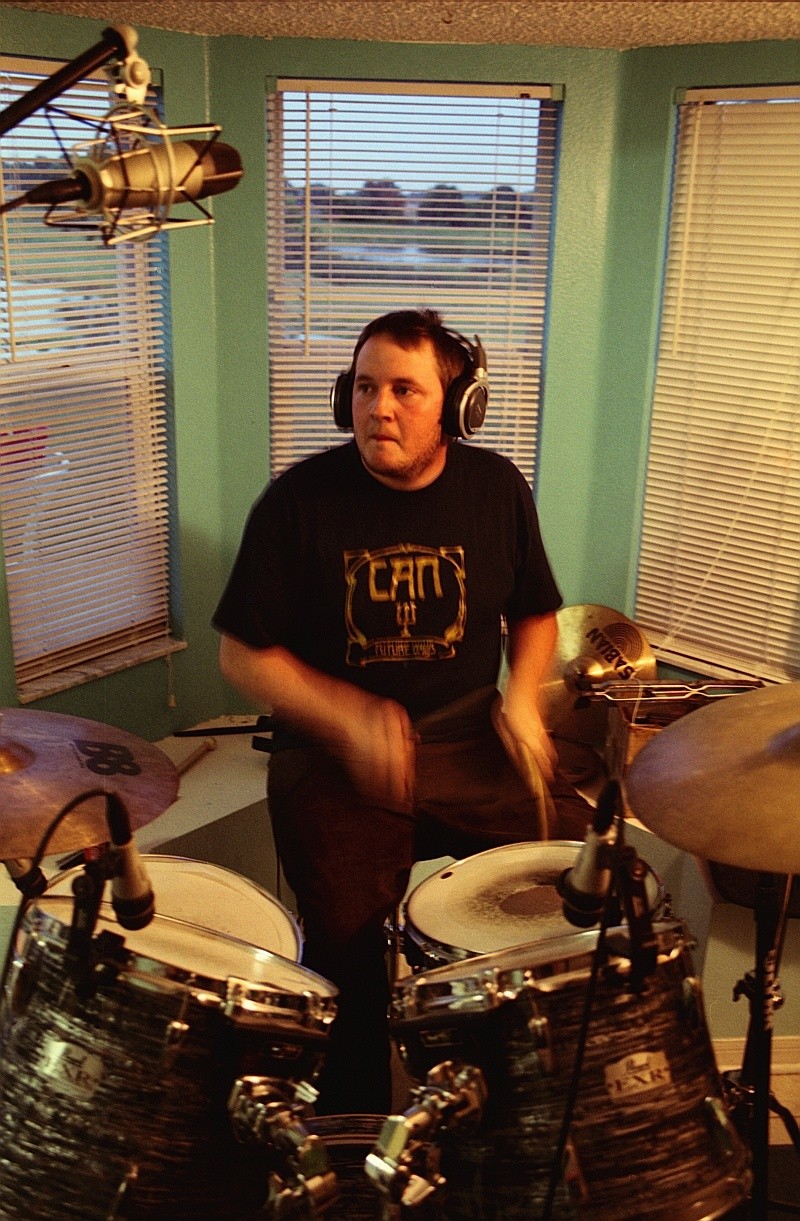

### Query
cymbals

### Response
[
  {"left": 535, "top": 605, "right": 658, "bottom": 741},
  {"left": 2, "top": 705, "right": 179, "bottom": 859},
  {"left": 627, "top": 679, "right": 799, "bottom": 875}
]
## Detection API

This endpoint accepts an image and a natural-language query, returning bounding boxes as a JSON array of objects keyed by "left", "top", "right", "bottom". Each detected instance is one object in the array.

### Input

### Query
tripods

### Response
[{"left": 719, "top": 874, "right": 800, "bottom": 1221}]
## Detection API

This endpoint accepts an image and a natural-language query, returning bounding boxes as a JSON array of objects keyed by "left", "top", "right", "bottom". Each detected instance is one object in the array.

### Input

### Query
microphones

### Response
[
  {"left": 5, "top": 858, "right": 48, "bottom": 898},
  {"left": 553, "top": 780, "right": 620, "bottom": 927},
  {"left": 26, "top": 139, "right": 242, "bottom": 209},
  {"left": 103, "top": 790, "right": 155, "bottom": 931}
]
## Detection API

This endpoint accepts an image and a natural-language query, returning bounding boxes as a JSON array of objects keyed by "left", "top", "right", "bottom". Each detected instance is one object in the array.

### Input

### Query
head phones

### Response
[{"left": 329, "top": 326, "right": 490, "bottom": 441}]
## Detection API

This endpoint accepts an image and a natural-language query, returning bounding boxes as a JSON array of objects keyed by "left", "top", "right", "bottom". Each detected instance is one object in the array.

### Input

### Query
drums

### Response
[
  {"left": 402, "top": 841, "right": 664, "bottom": 976},
  {"left": 388, "top": 915, "right": 752, "bottom": 1221},
  {"left": 32, "top": 852, "right": 303, "bottom": 967},
  {"left": 0, "top": 897, "right": 341, "bottom": 1221}
]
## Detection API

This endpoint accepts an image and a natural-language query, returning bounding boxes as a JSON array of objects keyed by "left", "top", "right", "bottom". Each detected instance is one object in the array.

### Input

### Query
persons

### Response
[{"left": 208, "top": 308, "right": 598, "bottom": 1221}]
